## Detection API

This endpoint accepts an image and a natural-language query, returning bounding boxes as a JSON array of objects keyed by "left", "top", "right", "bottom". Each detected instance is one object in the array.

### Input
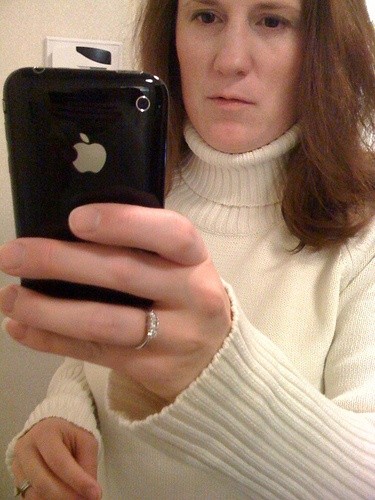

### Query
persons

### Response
[{"left": 2, "top": 1, "right": 375, "bottom": 500}]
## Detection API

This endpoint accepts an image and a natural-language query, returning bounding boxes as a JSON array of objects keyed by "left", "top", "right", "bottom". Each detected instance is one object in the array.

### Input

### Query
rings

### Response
[
  {"left": 12, "top": 478, "right": 33, "bottom": 499},
  {"left": 133, "top": 305, "right": 159, "bottom": 351}
]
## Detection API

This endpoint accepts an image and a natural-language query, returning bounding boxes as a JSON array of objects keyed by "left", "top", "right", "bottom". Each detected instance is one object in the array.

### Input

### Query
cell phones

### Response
[{"left": 3, "top": 68, "right": 168, "bottom": 311}]
